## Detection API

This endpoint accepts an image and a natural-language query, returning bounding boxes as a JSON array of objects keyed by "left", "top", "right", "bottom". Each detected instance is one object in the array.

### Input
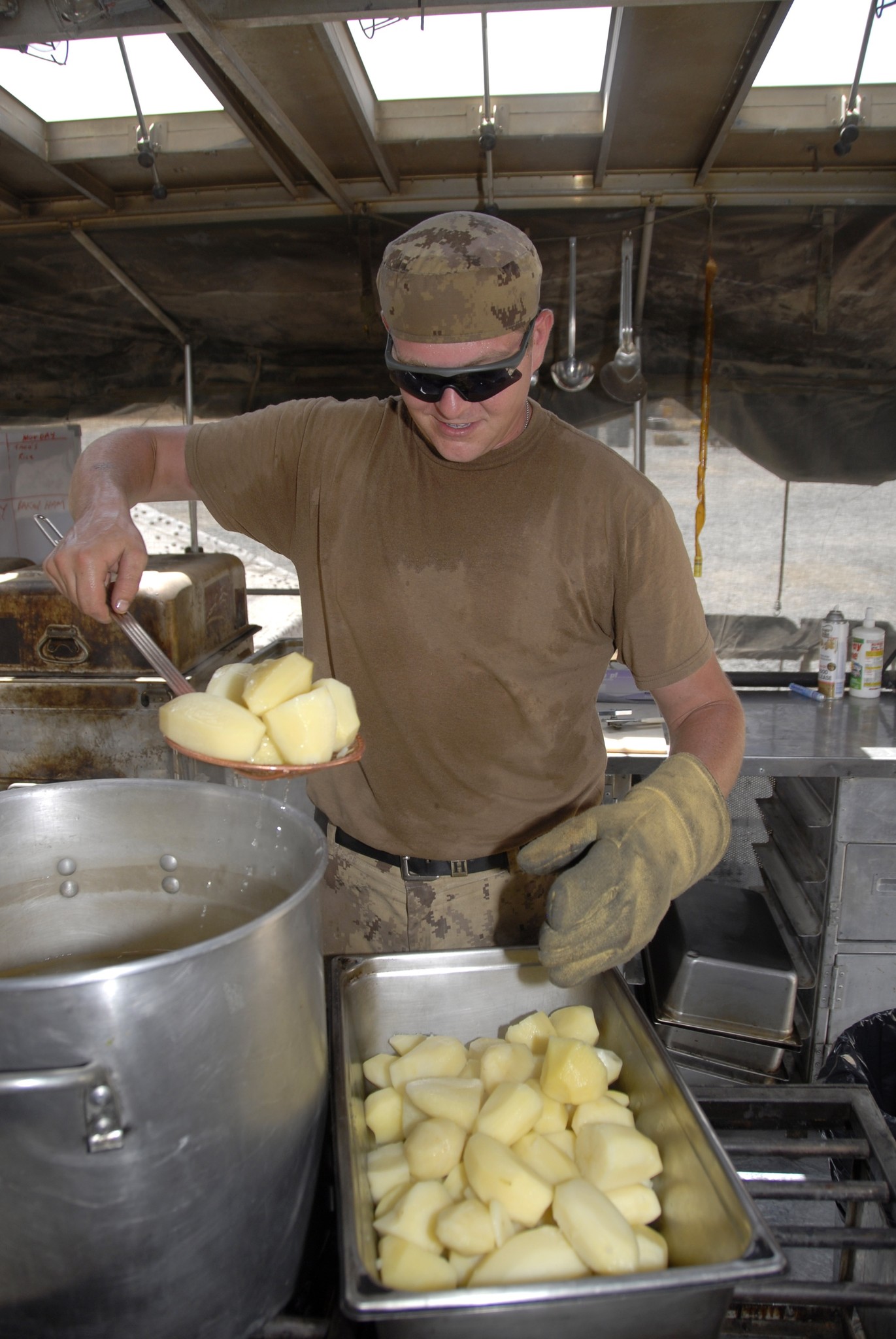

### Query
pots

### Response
[{"left": 3, "top": 778, "right": 331, "bottom": 1338}]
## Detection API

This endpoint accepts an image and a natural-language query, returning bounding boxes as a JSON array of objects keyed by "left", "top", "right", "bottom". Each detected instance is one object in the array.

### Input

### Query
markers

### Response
[{"left": 788, "top": 683, "right": 826, "bottom": 703}]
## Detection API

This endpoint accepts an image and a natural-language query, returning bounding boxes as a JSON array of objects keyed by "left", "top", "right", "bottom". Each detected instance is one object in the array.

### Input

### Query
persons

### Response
[{"left": 39, "top": 211, "right": 746, "bottom": 980}]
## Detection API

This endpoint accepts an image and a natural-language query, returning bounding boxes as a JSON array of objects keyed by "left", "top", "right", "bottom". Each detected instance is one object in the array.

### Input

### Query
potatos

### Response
[
  {"left": 158, "top": 652, "right": 361, "bottom": 765},
  {"left": 360, "top": 1006, "right": 671, "bottom": 1289}
]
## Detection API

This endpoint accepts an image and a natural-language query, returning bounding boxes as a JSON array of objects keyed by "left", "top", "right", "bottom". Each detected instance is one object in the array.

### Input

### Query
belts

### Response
[{"left": 314, "top": 806, "right": 537, "bottom": 882}]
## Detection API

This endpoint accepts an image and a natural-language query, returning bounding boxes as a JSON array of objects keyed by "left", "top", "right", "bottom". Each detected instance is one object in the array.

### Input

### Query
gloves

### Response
[{"left": 518, "top": 749, "right": 733, "bottom": 988}]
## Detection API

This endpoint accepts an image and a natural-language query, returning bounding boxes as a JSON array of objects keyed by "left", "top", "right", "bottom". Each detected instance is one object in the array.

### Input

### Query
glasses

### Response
[{"left": 385, "top": 309, "right": 542, "bottom": 403}]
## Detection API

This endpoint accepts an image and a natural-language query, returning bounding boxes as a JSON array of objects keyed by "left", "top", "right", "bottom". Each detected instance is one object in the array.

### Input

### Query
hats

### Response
[{"left": 376, "top": 211, "right": 543, "bottom": 345}]
